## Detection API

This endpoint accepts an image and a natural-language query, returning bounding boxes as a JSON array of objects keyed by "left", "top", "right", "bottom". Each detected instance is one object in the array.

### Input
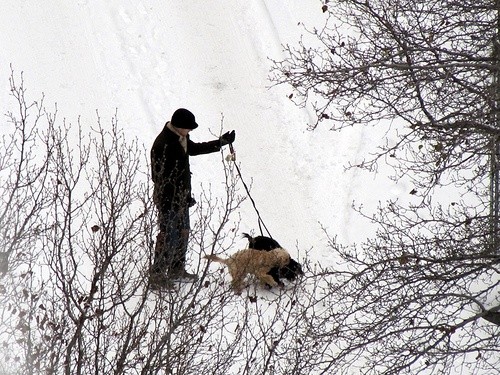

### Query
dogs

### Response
[
  {"left": 240, "top": 232, "right": 305, "bottom": 287},
  {"left": 203, "top": 248, "right": 290, "bottom": 296}
]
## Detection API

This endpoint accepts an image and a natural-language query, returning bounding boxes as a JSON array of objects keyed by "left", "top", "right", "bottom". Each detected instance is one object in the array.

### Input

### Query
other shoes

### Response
[
  {"left": 167, "top": 270, "right": 199, "bottom": 283},
  {"left": 149, "top": 275, "right": 177, "bottom": 290}
]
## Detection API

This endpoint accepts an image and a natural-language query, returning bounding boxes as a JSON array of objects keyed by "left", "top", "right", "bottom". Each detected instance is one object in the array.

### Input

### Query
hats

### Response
[{"left": 171, "top": 108, "right": 198, "bottom": 129}]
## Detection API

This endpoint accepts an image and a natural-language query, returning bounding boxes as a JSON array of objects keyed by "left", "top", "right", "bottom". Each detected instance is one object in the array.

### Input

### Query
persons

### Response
[{"left": 151, "top": 108, "right": 236, "bottom": 292}]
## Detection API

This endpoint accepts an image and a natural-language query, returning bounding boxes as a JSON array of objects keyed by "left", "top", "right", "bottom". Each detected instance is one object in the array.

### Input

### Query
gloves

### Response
[
  {"left": 188, "top": 197, "right": 196, "bottom": 207},
  {"left": 219, "top": 130, "right": 236, "bottom": 145}
]
